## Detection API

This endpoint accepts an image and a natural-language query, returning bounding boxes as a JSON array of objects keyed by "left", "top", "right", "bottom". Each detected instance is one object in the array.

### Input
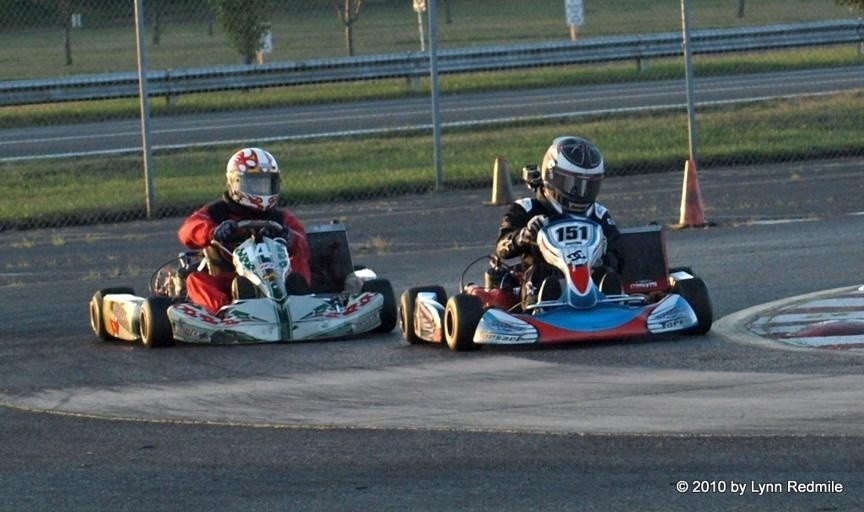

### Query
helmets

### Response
[
  {"left": 541, "top": 136, "right": 605, "bottom": 213},
  {"left": 226, "top": 148, "right": 282, "bottom": 210}
]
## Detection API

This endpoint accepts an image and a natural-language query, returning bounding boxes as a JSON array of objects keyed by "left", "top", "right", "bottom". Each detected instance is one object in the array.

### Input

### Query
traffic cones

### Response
[
  {"left": 482, "top": 154, "right": 518, "bottom": 206},
  {"left": 665, "top": 160, "right": 719, "bottom": 231}
]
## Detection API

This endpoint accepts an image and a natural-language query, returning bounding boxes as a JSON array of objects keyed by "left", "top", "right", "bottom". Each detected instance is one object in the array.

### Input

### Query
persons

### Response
[
  {"left": 497, "top": 135, "right": 628, "bottom": 313},
  {"left": 175, "top": 147, "right": 314, "bottom": 318}
]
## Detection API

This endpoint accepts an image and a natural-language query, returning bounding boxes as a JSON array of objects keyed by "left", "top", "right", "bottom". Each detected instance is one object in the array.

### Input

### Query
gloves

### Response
[
  {"left": 602, "top": 252, "right": 621, "bottom": 273},
  {"left": 276, "top": 222, "right": 294, "bottom": 247},
  {"left": 523, "top": 215, "right": 550, "bottom": 240},
  {"left": 214, "top": 219, "right": 238, "bottom": 242}
]
